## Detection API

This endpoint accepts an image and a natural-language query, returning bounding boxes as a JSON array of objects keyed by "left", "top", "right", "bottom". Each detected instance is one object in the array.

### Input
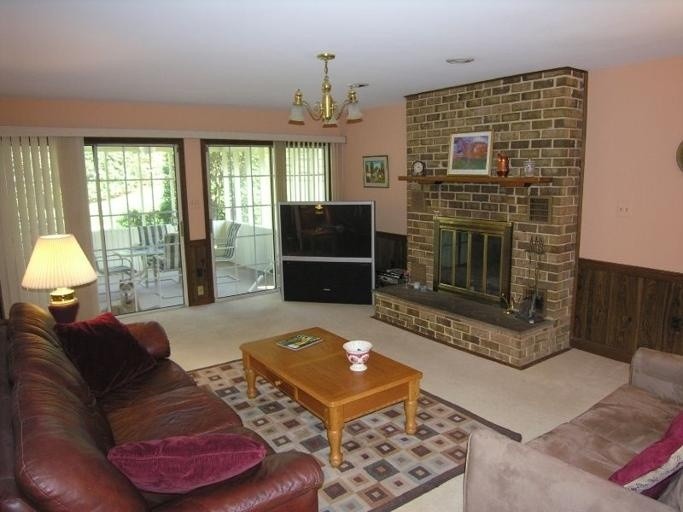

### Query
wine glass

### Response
[{"left": 343, "top": 340, "right": 373, "bottom": 372}]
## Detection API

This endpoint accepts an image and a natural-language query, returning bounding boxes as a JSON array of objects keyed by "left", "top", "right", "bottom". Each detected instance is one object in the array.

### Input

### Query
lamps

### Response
[
  {"left": 287, "top": 51, "right": 363, "bottom": 129},
  {"left": 20, "top": 233, "right": 99, "bottom": 323}
]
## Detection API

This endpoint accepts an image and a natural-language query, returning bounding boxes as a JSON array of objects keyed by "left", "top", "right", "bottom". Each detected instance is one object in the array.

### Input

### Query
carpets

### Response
[{"left": 184, "top": 354, "right": 522, "bottom": 512}]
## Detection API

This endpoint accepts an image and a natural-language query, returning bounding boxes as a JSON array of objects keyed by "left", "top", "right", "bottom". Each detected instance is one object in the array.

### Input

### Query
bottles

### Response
[{"left": 496, "top": 153, "right": 509, "bottom": 177}]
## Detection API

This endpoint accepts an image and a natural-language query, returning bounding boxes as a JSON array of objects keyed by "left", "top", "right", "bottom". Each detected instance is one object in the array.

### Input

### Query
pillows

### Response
[
  {"left": 106, "top": 434, "right": 267, "bottom": 495},
  {"left": 608, "top": 411, "right": 681, "bottom": 502},
  {"left": 54, "top": 313, "right": 160, "bottom": 394}
]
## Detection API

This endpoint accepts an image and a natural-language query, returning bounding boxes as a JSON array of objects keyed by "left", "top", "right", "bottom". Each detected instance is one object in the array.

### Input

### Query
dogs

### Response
[
  {"left": 100, "top": 282, "right": 136, "bottom": 315},
  {"left": 147, "top": 256, "right": 165, "bottom": 271}
]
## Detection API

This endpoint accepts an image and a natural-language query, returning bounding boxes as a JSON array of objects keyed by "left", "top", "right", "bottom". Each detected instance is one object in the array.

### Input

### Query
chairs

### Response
[{"left": 91, "top": 222, "right": 243, "bottom": 299}]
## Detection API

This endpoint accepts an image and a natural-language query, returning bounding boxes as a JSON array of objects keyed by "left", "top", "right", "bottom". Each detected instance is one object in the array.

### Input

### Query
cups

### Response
[{"left": 523, "top": 161, "right": 536, "bottom": 177}]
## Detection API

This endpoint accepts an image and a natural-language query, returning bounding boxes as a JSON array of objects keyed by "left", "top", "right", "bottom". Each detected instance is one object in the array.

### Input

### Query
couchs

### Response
[
  {"left": 1, "top": 301, "right": 325, "bottom": 512},
  {"left": 461, "top": 347, "right": 682, "bottom": 510}
]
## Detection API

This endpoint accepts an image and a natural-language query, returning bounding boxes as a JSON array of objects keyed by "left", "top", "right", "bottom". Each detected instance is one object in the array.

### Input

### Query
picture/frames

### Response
[
  {"left": 360, "top": 154, "right": 389, "bottom": 189},
  {"left": 446, "top": 130, "right": 494, "bottom": 177}
]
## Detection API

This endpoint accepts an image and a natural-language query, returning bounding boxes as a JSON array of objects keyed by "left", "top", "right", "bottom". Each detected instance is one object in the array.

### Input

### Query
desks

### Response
[{"left": 298, "top": 228, "right": 336, "bottom": 257}]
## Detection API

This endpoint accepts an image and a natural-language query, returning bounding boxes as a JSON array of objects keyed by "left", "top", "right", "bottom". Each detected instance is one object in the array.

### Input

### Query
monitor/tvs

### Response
[{"left": 278, "top": 200, "right": 376, "bottom": 259}]
